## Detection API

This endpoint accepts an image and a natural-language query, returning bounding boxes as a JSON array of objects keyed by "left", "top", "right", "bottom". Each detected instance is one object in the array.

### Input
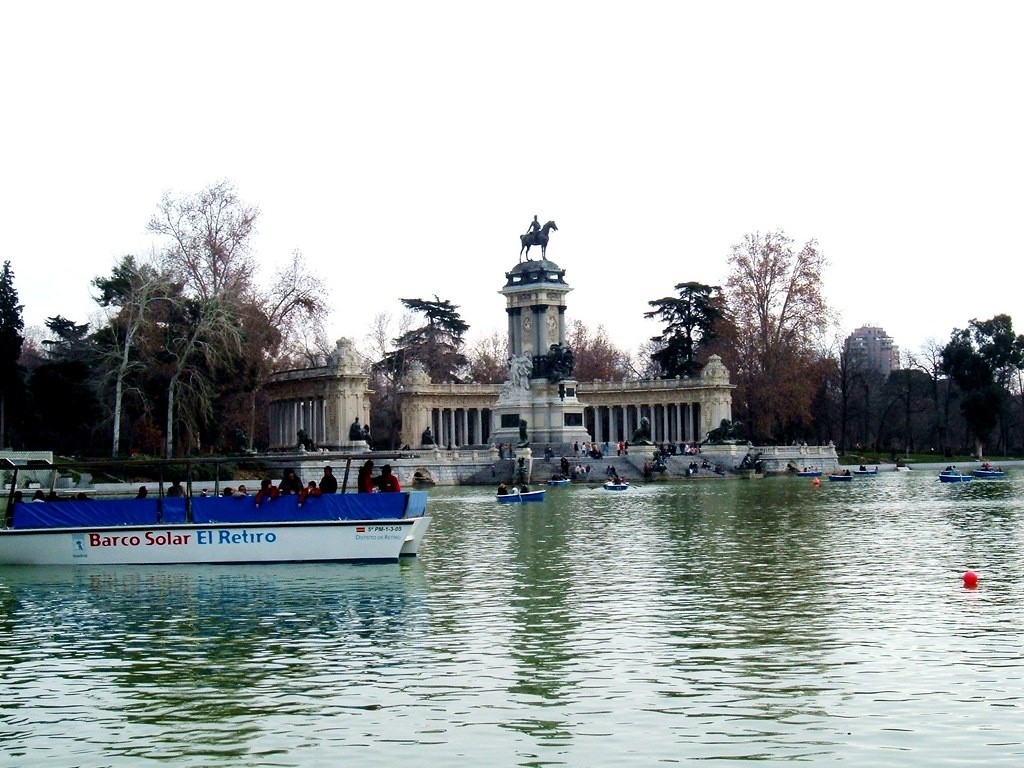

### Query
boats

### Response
[
  {"left": 797, "top": 471, "right": 823, "bottom": 477},
  {"left": 939, "top": 470, "right": 973, "bottom": 482},
  {"left": 972, "top": 467, "right": 1005, "bottom": 477},
  {"left": 0, "top": 452, "right": 435, "bottom": 564},
  {"left": 494, "top": 490, "right": 547, "bottom": 501},
  {"left": 603, "top": 481, "right": 630, "bottom": 488},
  {"left": 828, "top": 475, "right": 854, "bottom": 481},
  {"left": 605, "top": 483, "right": 627, "bottom": 490},
  {"left": 853, "top": 469, "right": 877, "bottom": 475},
  {"left": 547, "top": 479, "right": 570, "bottom": 486}
]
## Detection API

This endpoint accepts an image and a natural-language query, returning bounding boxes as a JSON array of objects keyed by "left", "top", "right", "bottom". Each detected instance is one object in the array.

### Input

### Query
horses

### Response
[{"left": 519, "top": 221, "right": 558, "bottom": 263}]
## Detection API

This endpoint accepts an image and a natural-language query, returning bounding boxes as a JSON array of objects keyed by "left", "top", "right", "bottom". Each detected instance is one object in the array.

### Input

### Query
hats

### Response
[{"left": 380, "top": 464, "right": 390, "bottom": 470}]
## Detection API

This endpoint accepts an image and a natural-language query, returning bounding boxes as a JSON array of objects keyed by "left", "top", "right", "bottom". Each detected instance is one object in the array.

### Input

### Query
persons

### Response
[
  {"left": 527, "top": 214, "right": 540, "bottom": 244},
  {"left": 643, "top": 462, "right": 649, "bottom": 477},
  {"left": 371, "top": 464, "right": 401, "bottom": 492},
  {"left": 791, "top": 439, "right": 808, "bottom": 447},
  {"left": 298, "top": 480, "right": 321, "bottom": 508},
  {"left": 318, "top": 466, "right": 338, "bottom": 494},
  {"left": 982, "top": 462, "right": 989, "bottom": 471},
  {"left": 996, "top": 464, "right": 1002, "bottom": 472},
  {"left": 945, "top": 465, "right": 957, "bottom": 471},
  {"left": 134, "top": 468, "right": 305, "bottom": 509},
  {"left": 349, "top": 417, "right": 362, "bottom": 440},
  {"left": 652, "top": 440, "right": 726, "bottom": 477},
  {"left": 495, "top": 481, "right": 529, "bottom": 496},
  {"left": 498, "top": 442, "right": 513, "bottom": 459},
  {"left": 544, "top": 440, "right": 630, "bottom": 485},
  {"left": 832, "top": 471, "right": 838, "bottom": 475},
  {"left": 804, "top": 465, "right": 817, "bottom": 472},
  {"left": 422, "top": 427, "right": 434, "bottom": 444},
  {"left": 357, "top": 460, "right": 373, "bottom": 493},
  {"left": 840, "top": 468, "right": 851, "bottom": 476},
  {"left": 297, "top": 429, "right": 317, "bottom": 451},
  {"left": 6, "top": 490, "right": 94, "bottom": 529},
  {"left": 860, "top": 464, "right": 866, "bottom": 471}
]
